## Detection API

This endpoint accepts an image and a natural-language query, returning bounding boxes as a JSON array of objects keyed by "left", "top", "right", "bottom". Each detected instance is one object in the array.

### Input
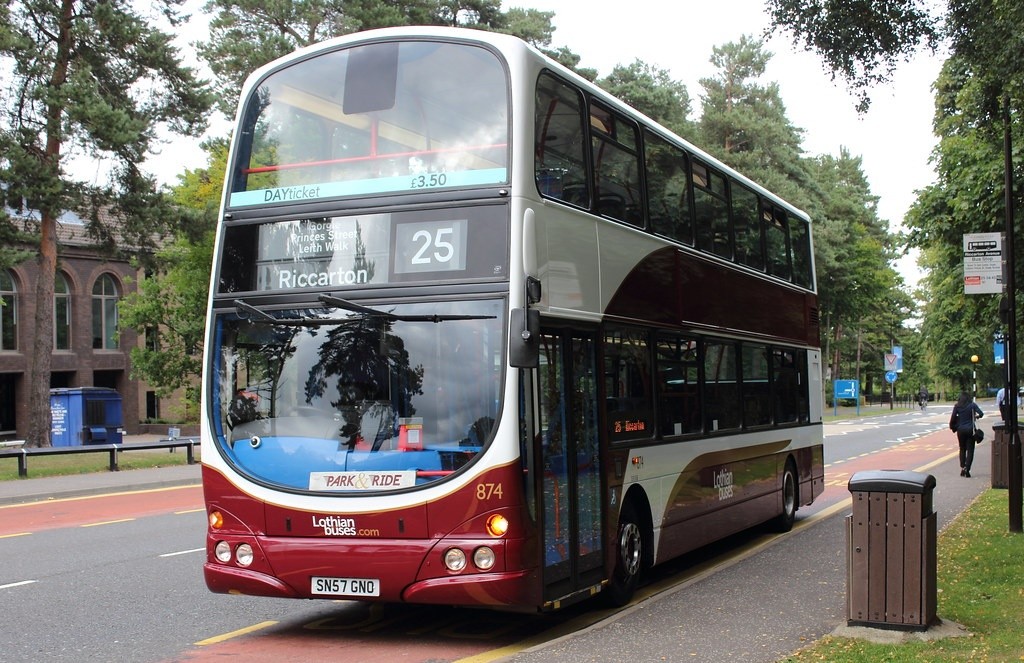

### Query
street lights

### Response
[{"left": 970, "top": 354, "right": 979, "bottom": 404}]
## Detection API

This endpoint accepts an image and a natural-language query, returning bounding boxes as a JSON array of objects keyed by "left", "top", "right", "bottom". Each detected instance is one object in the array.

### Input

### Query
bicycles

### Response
[{"left": 920, "top": 397, "right": 927, "bottom": 411}]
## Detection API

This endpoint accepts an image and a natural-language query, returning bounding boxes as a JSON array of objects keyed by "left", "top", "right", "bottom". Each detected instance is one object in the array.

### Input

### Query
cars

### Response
[{"left": 1018, "top": 386, "right": 1024, "bottom": 397}]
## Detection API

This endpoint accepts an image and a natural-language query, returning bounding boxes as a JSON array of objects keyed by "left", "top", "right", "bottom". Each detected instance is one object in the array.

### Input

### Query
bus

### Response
[{"left": 202, "top": 26, "right": 827, "bottom": 613}]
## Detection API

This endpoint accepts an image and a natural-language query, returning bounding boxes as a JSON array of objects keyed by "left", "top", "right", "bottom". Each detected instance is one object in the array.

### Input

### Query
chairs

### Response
[
  {"left": 559, "top": 182, "right": 812, "bottom": 289},
  {"left": 604, "top": 385, "right": 800, "bottom": 441}
]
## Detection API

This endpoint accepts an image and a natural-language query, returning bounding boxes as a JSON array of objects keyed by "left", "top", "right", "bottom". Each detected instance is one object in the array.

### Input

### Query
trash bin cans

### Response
[
  {"left": 844, "top": 469, "right": 938, "bottom": 630},
  {"left": 991, "top": 421, "right": 1024, "bottom": 489}
]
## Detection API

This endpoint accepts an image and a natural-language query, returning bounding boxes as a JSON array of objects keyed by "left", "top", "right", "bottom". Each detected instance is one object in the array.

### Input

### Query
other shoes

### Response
[
  {"left": 964, "top": 467, "right": 971, "bottom": 478},
  {"left": 960, "top": 468, "right": 965, "bottom": 477}
]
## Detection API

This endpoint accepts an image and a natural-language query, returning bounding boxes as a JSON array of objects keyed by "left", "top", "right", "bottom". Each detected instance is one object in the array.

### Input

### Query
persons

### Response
[
  {"left": 918, "top": 384, "right": 928, "bottom": 412},
  {"left": 996, "top": 385, "right": 1012, "bottom": 421},
  {"left": 950, "top": 392, "right": 984, "bottom": 477}
]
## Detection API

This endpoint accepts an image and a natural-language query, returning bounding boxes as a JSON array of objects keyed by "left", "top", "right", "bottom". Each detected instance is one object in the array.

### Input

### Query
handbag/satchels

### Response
[{"left": 973, "top": 429, "right": 984, "bottom": 443}]
